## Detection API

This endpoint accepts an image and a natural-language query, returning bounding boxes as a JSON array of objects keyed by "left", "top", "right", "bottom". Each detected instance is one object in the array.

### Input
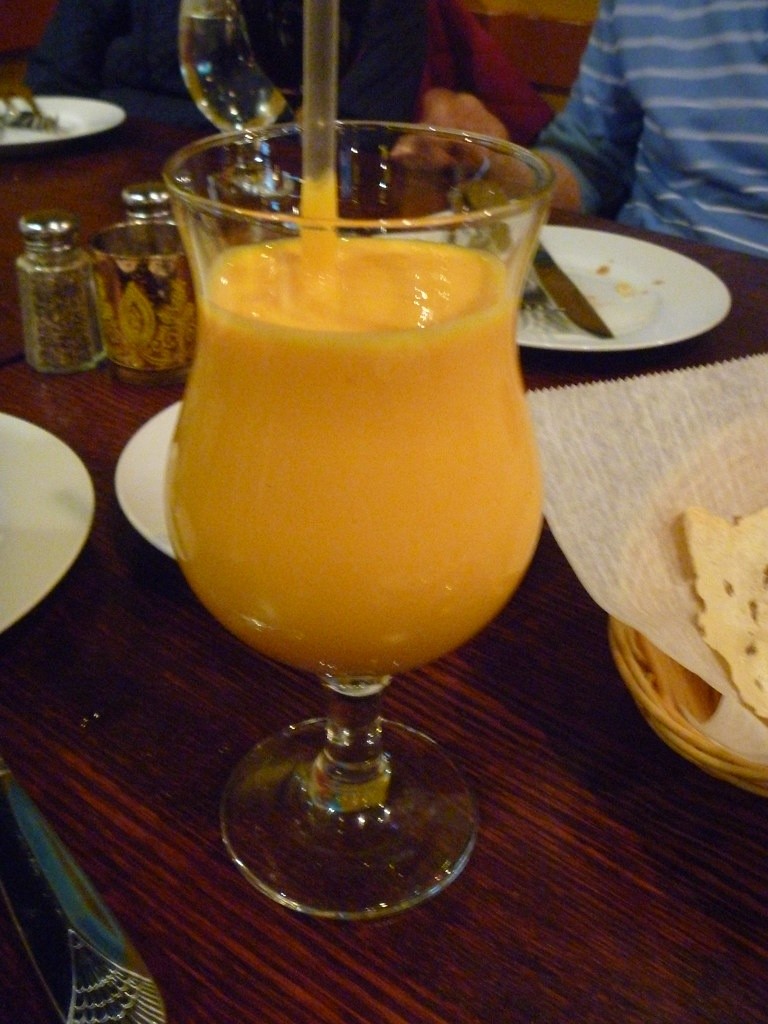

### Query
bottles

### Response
[
  {"left": 14, "top": 210, "right": 106, "bottom": 376},
  {"left": 121, "top": 182, "right": 177, "bottom": 223}
]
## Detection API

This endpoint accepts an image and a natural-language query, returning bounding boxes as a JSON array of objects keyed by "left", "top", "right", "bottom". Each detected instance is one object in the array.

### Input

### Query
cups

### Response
[
  {"left": 154, "top": 119, "right": 556, "bottom": 920},
  {"left": 179, "top": 0, "right": 293, "bottom": 198},
  {"left": 87, "top": 220, "right": 197, "bottom": 388}
]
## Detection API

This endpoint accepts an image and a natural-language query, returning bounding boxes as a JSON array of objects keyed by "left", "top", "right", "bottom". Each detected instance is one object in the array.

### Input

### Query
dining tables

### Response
[{"left": 0, "top": 83, "right": 767, "bottom": 1024}]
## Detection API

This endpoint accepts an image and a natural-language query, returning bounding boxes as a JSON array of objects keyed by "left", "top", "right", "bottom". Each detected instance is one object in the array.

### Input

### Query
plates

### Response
[
  {"left": 0, "top": 409, "right": 96, "bottom": 636},
  {"left": 0, "top": 94, "right": 126, "bottom": 147},
  {"left": 368, "top": 221, "right": 731, "bottom": 351},
  {"left": 116, "top": 400, "right": 179, "bottom": 561}
]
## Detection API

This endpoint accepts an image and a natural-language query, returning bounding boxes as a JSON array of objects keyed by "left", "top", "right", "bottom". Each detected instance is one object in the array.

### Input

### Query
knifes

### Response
[{"left": 447, "top": 142, "right": 617, "bottom": 338}]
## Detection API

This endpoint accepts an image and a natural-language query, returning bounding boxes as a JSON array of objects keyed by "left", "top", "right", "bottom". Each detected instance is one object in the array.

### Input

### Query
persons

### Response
[{"left": 0, "top": 1, "right": 768, "bottom": 263}]
